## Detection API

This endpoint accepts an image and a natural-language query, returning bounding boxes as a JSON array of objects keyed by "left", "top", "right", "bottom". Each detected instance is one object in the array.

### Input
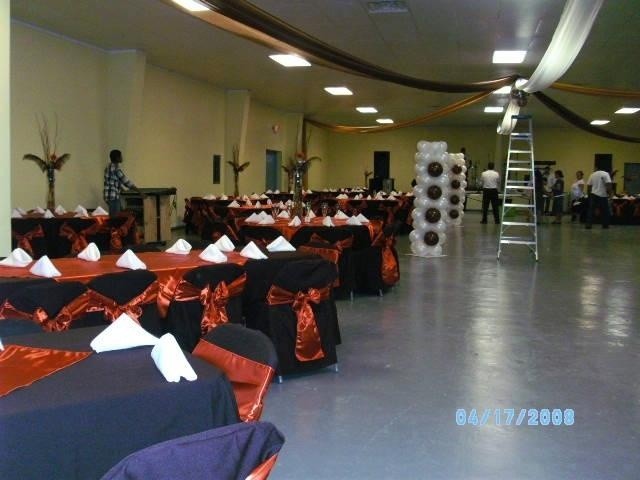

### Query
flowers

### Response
[
  {"left": 225, "top": 143, "right": 250, "bottom": 197},
  {"left": 280, "top": 115, "right": 321, "bottom": 218},
  {"left": 22, "top": 111, "right": 72, "bottom": 209}
]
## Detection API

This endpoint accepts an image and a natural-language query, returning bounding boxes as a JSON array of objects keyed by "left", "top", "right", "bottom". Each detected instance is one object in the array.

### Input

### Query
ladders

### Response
[{"left": 496, "top": 115, "right": 538, "bottom": 261}]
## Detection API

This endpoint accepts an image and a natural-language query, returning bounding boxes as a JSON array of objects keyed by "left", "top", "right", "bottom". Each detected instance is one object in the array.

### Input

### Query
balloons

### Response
[
  {"left": 407, "top": 140, "right": 468, "bottom": 258},
  {"left": 510, "top": 83, "right": 532, "bottom": 107}
]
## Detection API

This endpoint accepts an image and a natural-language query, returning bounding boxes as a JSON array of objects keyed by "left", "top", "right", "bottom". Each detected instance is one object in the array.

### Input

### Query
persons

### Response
[
  {"left": 478, "top": 161, "right": 502, "bottom": 225},
  {"left": 460, "top": 147, "right": 473, "bottom": 191},
  {"left": 522, "top": 162, "right": 614, "bottom": 231},
  {"left": 103, "top": 149, "right": 147, "bottom": 218}
]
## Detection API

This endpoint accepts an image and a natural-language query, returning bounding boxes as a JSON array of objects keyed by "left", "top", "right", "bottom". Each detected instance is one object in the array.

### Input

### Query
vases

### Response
[
  {"left": 233, "top": 175, "right": 239, "bottom": 197},
  {"left": 46, "top": 178, "right": 56, "bottom": 213},
  {"left": 291, "top": 167, "right": 303, "bottom": 219}
]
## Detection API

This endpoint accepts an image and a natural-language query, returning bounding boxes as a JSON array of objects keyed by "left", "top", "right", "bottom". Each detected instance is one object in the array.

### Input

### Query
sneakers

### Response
[
  {"left": 552, "top": 220, "right": 561, "bottom": 224},
  {"left": 480, "top": 220, "right": 487, "bottom": 223},
  {"left": 602, "top": 225, "right": 609, "bottom": 228},
  {"left": 495, "top": 221, "right": 500, "bottom": 224}
]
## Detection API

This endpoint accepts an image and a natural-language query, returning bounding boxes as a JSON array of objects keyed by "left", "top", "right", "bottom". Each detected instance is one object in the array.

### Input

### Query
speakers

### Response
[
  {"left": 594, "top": 153, "right": 612, "bottom": 176},
  {"left": 373, "top": 150, "right": 390, "bottom": 180}
]
